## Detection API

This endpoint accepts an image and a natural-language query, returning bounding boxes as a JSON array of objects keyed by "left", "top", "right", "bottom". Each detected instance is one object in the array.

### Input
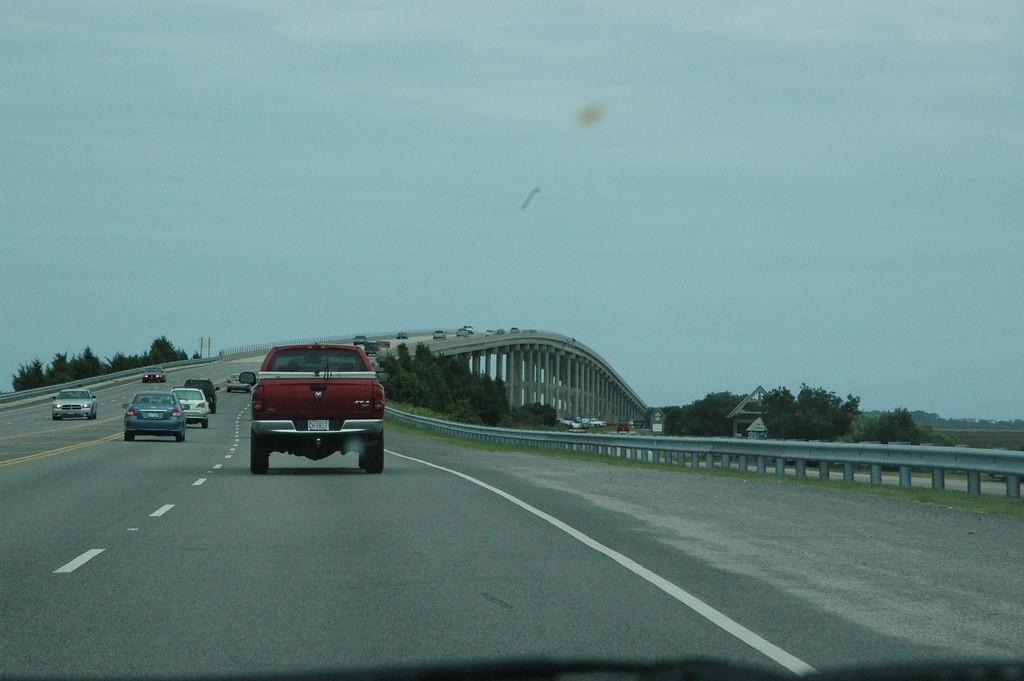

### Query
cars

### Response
[
  {"left": 353, "top": 335, "right": 368, "bottom": 346},
  {"left": 122, "top": 391, "right": 191, "bottom": 442},
  {"left": 226, "top": 373, "right": 251, "bottom": 393},
  {"left": 432, "top": 329, "right": 449, "bottom": 341},
  {"left": 455, "top": 325, "right": 475, "bottom": 337},
  {"left": 484, "top": 327, "right": 520, "bottom": 337},
  {"left": 396, "top": 331, "right": 408, "bottom": 340},
  {"left": 141, "top": 367, "right": 166, "bottom": 383},
  {"left": 52, "top": 389, "right": 98, "bottom": 421},
  {"left": 560, "top": 418, "right": 608, "bottom": 429}
]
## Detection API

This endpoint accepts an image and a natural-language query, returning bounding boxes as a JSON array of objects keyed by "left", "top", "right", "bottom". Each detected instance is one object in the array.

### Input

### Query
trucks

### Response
[{"left": 617, "top": 419, "right": 630, "bottom": 433}]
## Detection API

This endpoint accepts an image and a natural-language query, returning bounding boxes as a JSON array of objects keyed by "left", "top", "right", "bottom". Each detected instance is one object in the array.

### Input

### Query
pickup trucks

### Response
[{"left": 239, "top": 341, "right": 391, "bottom": 476}]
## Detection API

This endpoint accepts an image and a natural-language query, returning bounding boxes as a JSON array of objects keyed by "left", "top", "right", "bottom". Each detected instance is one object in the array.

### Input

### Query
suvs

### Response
[
  {"left": 168, "top": 386, "right": 213, "bottom": 429},
  {"left": 184, "top": 377, "right": 220, "bottom": 414}
]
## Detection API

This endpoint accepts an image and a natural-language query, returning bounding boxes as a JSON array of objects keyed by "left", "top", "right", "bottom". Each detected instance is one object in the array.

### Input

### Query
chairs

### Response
[
  {"left": 162, "top": 400, "right": 171, "bottom": 404},
  {"left": 337, "top": 362, "right": 356, "bottom": 372},
  {"left": 81, "top": 392, "right": 90, "bottom": 399},
  {"left": 278, "top": 366, "right": 300, "bottom": 372},
  {"left": 62, "top": 394, "right": 70, "bottom": 399},
  {"left": 139, "top": 397, "right": 150, "bottom": 404}
]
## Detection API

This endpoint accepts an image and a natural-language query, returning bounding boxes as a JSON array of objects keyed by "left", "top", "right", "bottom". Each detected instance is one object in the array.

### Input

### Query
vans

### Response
[{"left": 365, "top": 342, "right": 378, "bottom": 356}]
